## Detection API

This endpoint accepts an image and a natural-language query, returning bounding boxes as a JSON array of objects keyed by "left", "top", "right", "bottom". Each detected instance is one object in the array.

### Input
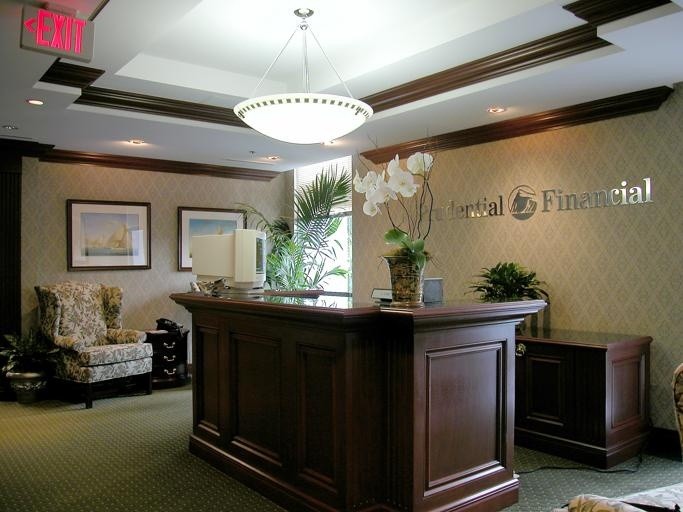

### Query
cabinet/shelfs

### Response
[
  {"left": 146, "top": 329, "right": 192, "bottom": 391},
  {"left": 508, "top": 325, "right": 654, "bottom": 468}
]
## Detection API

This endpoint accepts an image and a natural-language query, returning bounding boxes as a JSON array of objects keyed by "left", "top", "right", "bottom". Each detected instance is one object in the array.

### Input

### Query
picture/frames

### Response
[{"left": 65, "top": 198, "right": 248, "bottom": 272}]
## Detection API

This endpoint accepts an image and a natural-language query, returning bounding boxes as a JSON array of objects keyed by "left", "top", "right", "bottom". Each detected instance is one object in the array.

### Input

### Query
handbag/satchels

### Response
[{"left": 155, "top": 318, "right": 183, "bottom": 332}]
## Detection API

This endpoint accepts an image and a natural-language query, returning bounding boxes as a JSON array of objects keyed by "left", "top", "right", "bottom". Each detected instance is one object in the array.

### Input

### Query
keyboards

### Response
[{"left": 264, "top": 289, "right": 319, "bottom": 298}]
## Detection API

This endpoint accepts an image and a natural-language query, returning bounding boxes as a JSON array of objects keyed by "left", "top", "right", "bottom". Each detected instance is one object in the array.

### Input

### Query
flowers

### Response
[{"left": 355, "top": 129, "right": 440, "bottom": 272}]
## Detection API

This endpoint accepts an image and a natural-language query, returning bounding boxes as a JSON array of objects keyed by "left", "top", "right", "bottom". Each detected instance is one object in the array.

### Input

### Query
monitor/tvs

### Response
[{"left": 191, "top": 229, "right": 267, "bottom": 294}]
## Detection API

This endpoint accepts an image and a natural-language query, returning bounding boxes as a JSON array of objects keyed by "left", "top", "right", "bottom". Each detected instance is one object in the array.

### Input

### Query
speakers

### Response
[{"left": 423, "top": 277, "right": 442, "bottom": 303}]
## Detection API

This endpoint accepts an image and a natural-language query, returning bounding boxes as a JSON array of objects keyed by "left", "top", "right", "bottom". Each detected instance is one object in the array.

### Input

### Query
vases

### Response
[{"left": 382, "top": 250, "right": 433, "bottom": 309}]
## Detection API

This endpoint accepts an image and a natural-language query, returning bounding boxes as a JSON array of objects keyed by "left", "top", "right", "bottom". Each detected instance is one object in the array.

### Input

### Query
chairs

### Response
[{"left": 28, "top": 280, "right": 154, "bottom": 409}]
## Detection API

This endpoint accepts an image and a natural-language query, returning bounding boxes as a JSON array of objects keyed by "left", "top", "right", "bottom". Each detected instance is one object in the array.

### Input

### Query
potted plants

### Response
[{"left": 1, "top": 328, "right": 62, "bottom": 405}]
